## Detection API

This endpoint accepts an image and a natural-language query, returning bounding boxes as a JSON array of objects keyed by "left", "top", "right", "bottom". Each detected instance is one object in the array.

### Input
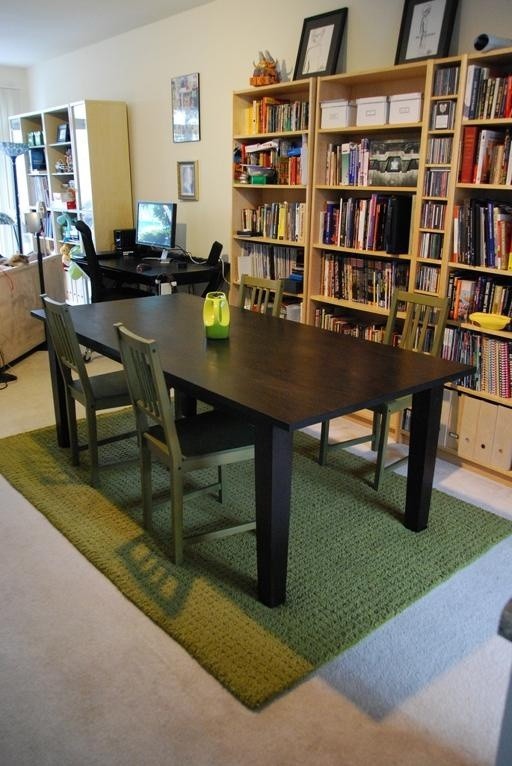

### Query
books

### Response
[
  {"left": 411, "top": 66, "right": 512, "bottom": 398},
  {"left": 238, "top": 97, "right": 304, "bottom": 324},
  {"left": 317, "top": 136, "right": 412, "bottom": 347},
  {"left": 31, "top": 175, "right": 53, "bottom": 238}
]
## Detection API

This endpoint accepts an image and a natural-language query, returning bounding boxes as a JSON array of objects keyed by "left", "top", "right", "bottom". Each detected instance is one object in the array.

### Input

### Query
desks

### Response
[
  {"left": 28, "top": 292, "right": 474, "bottom": 609},
  {"left": 69, "top": 250, "right": 228, "bottom": 303}
]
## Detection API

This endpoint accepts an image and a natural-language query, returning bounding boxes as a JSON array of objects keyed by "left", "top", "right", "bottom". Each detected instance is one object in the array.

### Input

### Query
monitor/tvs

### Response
[{"left": 135, "top": 201, "right": 177, "bottom": 263}]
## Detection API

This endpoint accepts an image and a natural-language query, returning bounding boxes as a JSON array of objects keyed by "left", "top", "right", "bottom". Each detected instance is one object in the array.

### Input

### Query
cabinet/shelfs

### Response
[{"left": 7, "top": 101, "right": 130, "bottom": 298}]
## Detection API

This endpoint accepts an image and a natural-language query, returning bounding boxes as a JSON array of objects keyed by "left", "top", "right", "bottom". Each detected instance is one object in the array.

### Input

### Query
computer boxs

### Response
[{"left": 114, "top": 229, "right": 136, "bottom": 253}]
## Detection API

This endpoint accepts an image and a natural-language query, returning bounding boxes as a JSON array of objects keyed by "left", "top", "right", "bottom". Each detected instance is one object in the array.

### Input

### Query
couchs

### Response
[{"left": 0, "top": 253, "right": 67, "bottom": 370}]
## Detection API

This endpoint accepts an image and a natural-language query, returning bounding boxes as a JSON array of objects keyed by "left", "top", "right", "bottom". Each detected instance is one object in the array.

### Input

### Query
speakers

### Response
[
  {"left": 207, "top": 241, "right": 223, "bottom": 266},
  {"left": 133, "top": 244, "right": 146, "bottom": 258}
]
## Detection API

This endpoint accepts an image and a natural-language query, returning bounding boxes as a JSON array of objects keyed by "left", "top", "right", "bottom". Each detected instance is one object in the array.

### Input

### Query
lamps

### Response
[{"left": 0, "top": 141, "right": 29, "bottom": 263}]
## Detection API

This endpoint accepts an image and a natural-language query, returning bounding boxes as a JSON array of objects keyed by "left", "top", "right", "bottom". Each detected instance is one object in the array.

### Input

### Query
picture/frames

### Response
[
  {"left": 176, "top": 160, "right": 199, "bottom": 199},
  {"left": 395, "top": 0, "right": 457, "bottom": 65},
  {"left": 292, "top": 7, "right": 349, "bottom": 76}
]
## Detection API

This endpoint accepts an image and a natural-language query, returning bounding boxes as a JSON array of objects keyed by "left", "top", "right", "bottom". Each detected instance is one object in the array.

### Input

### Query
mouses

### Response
[{"left": 137, "top": 264, "right": 151, "bottom": 269}]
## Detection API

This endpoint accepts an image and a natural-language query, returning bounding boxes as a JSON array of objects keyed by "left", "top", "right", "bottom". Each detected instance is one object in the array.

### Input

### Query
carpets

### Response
[{"left": 0, "top": 396, "right": 512, "bottom": 712}]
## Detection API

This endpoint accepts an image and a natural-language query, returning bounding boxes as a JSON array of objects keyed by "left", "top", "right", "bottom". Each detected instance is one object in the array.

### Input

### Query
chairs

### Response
[
  {"left": 40, "top": 295, "right": 131, "bottom": 484},
  {"left": 113, "top": 321, "right": 256, "bottom": 565},
  {"left": 75, "top": 221, "right": 158, "bottom": 362}
]
